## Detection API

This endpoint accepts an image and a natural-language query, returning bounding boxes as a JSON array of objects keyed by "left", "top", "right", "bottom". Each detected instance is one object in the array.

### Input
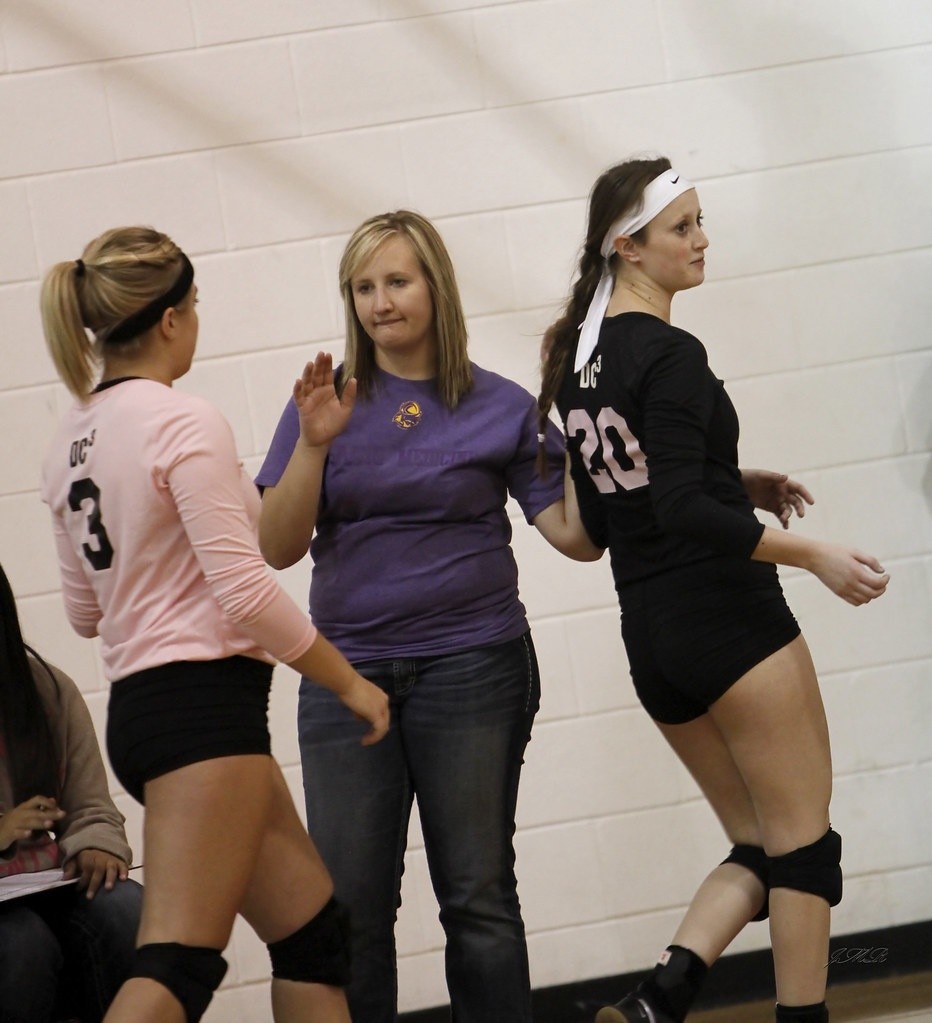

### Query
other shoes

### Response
[{"left": 595, "top": 981, "right": 674, "bottom": 1023}]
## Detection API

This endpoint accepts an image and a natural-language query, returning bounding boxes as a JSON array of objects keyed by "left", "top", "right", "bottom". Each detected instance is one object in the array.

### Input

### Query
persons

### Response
[
  {"left": 254, "top": 209, "right": 605, "bottom": 1023},
  {"left": 37, "top": 225, "right": 390, "bottom": 1023},
  {"left": 536, "top": 157, "right": 891, "bottom": 1023},
  {"left": 0, "top": 566, "right": 146, "bottom": 1023}
]
